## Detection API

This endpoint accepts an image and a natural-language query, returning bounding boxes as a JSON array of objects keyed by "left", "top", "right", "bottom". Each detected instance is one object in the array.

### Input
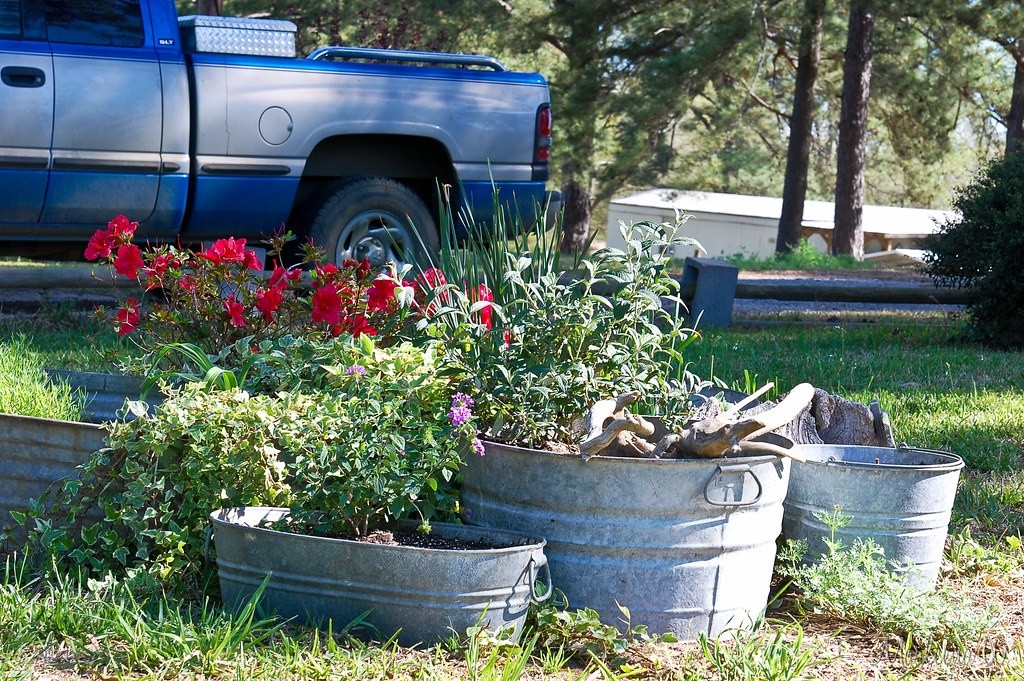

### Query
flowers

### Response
[
  {"left": 254, "top": 358, "right": 485, "bottom": 537},
  {"left": 84, "top": 214, "right": 513, "bottom": 376}
]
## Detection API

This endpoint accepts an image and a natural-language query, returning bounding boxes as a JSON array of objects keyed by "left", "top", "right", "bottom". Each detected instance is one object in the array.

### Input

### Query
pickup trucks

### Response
[{"left": 0, "top": 0, "right": 562, "bottom": 292}]
